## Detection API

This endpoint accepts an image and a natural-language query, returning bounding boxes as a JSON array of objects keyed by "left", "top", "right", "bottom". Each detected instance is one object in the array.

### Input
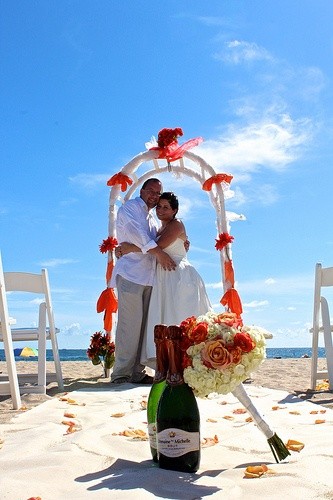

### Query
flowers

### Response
[
  {"left": 182, "top": 304, "right": 295, "bottom": 465},
  {"left": 85, "top": 329, "right": 116, "bottom": 368}
]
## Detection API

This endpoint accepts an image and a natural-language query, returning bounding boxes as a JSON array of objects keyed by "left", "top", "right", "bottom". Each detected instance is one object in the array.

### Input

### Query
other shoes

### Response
[
  {"left": 130, "top": 374, "right": 154, "bottom": 384},
  {"left": 114, "top": 376, "right": 129, "bottom": 384}
]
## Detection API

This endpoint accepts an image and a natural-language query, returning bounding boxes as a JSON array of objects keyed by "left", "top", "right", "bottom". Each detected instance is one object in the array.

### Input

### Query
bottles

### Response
[
  {"left": 147, "top": 324, "right": 167, "bottom": 463},
  {"left": 157, "top": 325, "right": 201, "bottom": 474}
]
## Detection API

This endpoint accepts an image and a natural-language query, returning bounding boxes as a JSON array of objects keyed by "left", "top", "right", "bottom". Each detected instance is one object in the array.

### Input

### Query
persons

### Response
[
  {"left": 111, "top": 178, "right": 190, "bottom": 384},
  {"left": 114, "top": 192, "right": 211, "bottom": 370}
]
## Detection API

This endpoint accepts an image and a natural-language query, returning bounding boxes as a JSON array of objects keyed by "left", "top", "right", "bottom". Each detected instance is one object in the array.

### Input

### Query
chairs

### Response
[
  {"left": 308, "top": 260, "right": 333, "bottom": 395},
  {"left": 0, "top": 252, "right": 22, "bottom": 410},
  {"left": 0, "top": 268, "right": 68, "bottom": 397}
]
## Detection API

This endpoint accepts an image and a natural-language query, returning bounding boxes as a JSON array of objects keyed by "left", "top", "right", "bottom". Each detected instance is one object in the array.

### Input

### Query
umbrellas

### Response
[{"left": 14, "top": 347, "right": 38, "bottom": 358}]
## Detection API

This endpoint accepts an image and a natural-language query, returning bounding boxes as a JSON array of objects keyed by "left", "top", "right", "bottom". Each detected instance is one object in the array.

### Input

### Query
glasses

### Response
[{"left": 141, "top": 189, "right": 162, "bottom": 196}]
